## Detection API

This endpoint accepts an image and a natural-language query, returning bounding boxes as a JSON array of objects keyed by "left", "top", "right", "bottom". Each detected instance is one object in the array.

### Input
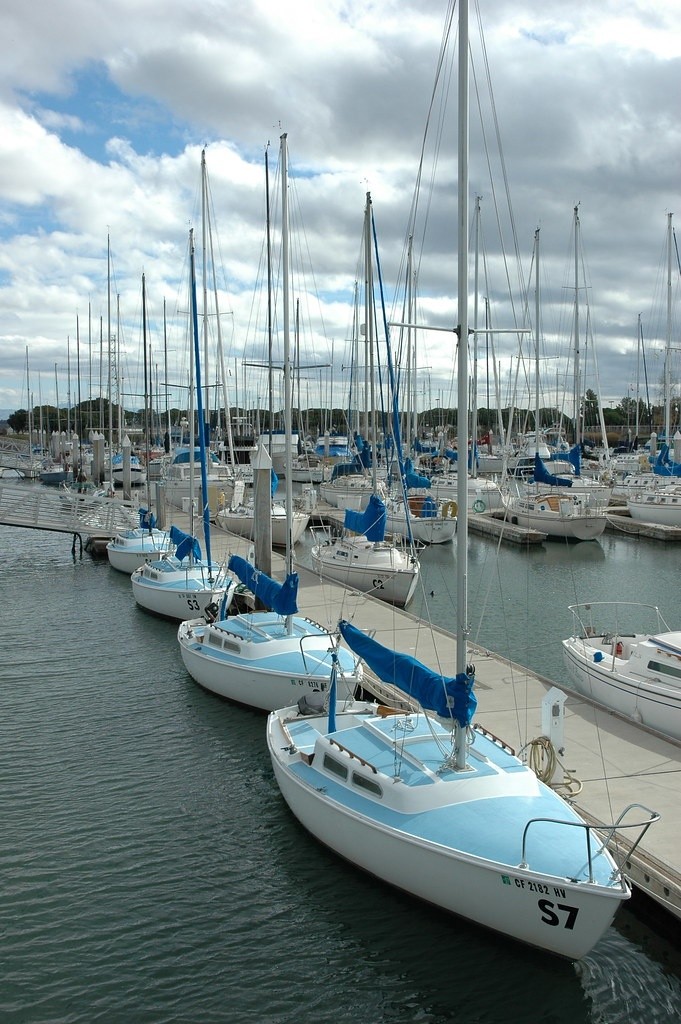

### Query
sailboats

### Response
[
  {"left": 174, "top": 127, "right": 368, "bottom": 712},
  {"left": 264, "top": 0, "right": 661, "bottom": 963},
  {"left": 1, "top": 191, "right": 681, "bottom": 619}
]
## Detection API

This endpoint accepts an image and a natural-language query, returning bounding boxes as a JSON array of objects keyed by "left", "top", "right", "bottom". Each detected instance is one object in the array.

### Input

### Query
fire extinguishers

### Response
[{"left": 617, "top": 642, "right": 624, "bottom": 655}]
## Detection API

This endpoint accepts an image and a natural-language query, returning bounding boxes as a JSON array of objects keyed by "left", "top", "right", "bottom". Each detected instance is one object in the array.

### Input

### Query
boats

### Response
[{"left": 560, "top": 601, "right": 680, "bottom": 743}]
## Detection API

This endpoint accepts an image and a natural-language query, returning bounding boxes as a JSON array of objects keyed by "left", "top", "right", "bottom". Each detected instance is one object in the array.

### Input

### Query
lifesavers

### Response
[
  {"left": 442, "top": 501, "right": 458, "bottom": 517},
  {"left": 599, "top": 471, "right": 612, "bottom": 485},
  {"left": 473, "top": 499, "right": 487, "bottom": 513}
]
